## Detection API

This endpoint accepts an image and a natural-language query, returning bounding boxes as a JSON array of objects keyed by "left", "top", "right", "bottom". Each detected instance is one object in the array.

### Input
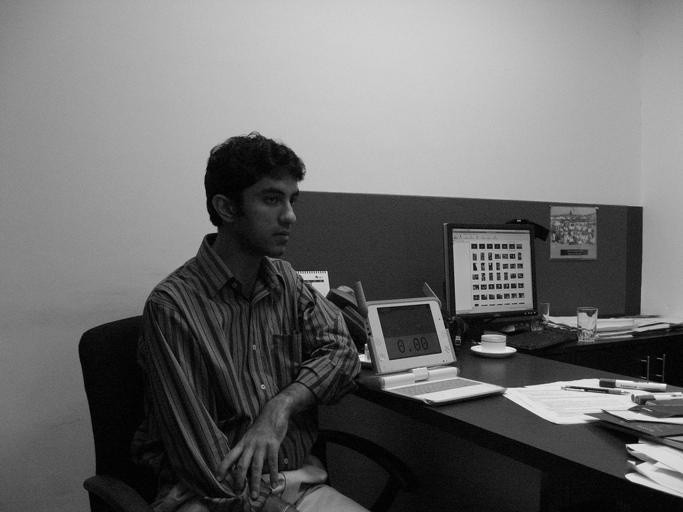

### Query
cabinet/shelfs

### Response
[{"left": 529, "top": 314, "right": 683, "bottom": 385}]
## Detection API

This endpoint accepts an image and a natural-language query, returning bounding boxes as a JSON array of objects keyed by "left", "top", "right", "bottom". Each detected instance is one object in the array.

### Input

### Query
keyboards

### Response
[{"left": 506, "top": 327, "right": 578, "bottom": 350}]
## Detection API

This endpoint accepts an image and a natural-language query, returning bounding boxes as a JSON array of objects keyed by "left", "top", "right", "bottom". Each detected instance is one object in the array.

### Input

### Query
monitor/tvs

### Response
[{"left": 443, "top": 222, "right": 538, "bottom": 342}]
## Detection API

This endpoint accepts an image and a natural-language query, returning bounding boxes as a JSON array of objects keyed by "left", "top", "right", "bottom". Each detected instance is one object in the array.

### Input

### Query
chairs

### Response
[{"left": 78, "top": 316, "right": 419, "bottom": 512}]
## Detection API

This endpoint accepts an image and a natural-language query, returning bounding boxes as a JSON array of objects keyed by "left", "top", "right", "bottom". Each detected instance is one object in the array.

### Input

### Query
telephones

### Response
[{"left": 327, "top": 284, "right": 369, "bottom": 354}]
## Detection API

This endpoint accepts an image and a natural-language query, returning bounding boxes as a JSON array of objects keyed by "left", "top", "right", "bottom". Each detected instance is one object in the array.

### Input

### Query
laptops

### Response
[{"left": 364, "top": 297, "right": 506, "bottom": 408}]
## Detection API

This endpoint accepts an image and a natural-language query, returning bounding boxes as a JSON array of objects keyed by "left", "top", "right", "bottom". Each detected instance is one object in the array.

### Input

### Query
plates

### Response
[{"left": 471, "top": 345, "right": 517, "bottom": 358}]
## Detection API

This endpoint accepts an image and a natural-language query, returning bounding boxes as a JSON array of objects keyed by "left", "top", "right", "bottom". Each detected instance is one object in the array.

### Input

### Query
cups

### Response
[
  {"left": 539, "top": 302, "right": 550, "bottom": 322},
  {"left": 577, "top": 306, "right": 598, "bottom": 342},
  {"left": 480, "top": 334, "right": 507, "bottom": 353}
]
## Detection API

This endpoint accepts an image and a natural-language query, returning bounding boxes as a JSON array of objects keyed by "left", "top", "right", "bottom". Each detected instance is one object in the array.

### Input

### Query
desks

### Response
[{"left": 351, "top": 348, "right": 683, "bottom": 512}]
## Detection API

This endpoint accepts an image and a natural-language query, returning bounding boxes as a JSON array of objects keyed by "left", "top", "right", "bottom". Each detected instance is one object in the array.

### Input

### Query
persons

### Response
[
  {"left": 549, "top": 213, "right": 596, "bottom": 246},
  {"left": 127, "top": 131, "right": 372, "bottom": 511}
]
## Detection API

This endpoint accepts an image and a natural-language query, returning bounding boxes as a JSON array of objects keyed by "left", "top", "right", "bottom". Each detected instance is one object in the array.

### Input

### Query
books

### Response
[{"left": 597, "top": 419, "right": 683, "bottom": 452}]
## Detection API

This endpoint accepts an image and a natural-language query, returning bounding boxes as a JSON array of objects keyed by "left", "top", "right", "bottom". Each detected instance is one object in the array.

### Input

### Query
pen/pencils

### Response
[{"left": 562, "top": 378, "right": 667, "bottom": 394}]
